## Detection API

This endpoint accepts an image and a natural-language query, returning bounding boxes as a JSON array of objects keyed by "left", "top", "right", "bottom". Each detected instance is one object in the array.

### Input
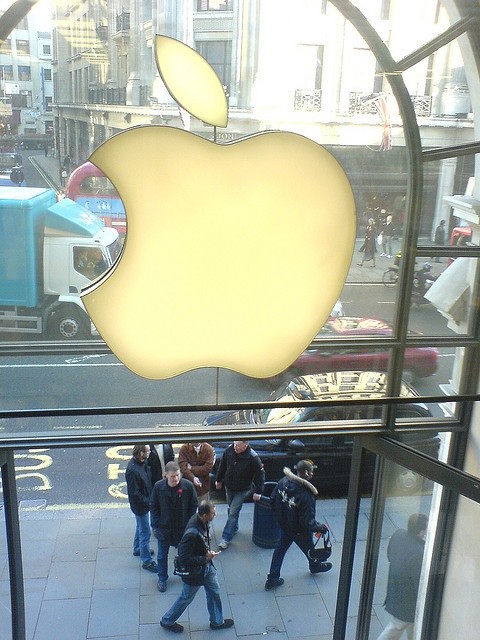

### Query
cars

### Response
[
  {"left": 200, "top": 369, "right": 443, "bottom": 501},
  {"left": 329, "top": 300, "right": 345, "bottom": 317},
  {"left": 247, "top": 315, "right": 439, "bottom": 381}
]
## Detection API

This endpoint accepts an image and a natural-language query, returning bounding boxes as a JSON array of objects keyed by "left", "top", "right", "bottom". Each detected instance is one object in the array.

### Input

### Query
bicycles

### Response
[{"left": 382, "top": 262, "right": 418, "bottom": 288}]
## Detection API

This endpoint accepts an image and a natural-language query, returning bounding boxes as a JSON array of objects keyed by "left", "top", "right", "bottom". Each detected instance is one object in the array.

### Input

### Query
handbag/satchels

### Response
[
  {"left": 307, "top": 528, "right": 333, "bottom": 564},
  {"left": 172, "top": 527, "right": 210, "bottom": 588}
]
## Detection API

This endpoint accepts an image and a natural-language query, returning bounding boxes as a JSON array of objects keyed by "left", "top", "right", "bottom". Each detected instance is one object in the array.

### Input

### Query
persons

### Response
[
  {"left": 150, "top": 461, "right": 198, "bottom": 592},
  {"left": 379, "top": 216, "right": 395, "bottom": 258},
  {"left": 376, "top": 513, "right": 429, "bottom": 640},
  {"left": 414, "top": 265, "right": 437, "bottom": 291},
  {"left": 376, "top": 209, "right": 387, "bottom": 235},
  {"left": 357, "top": 226, "right": 376, "bottom": 268},
  {"left": 64, "top": 155, "right": 70, "bottom": 174},
  {"left": 264, "top": 460, "right": 333, "bottom": 590},
  {"left": 147, "top": 443, "right": 175, "bottom": 488},
  {"left": 160, "top": 499, "right": 234, "bottom": 633},
  {"left": 44, "top": 142, "right": 49, "bottom": 157},
  {"left": 215, "top": 441, "right": 265, "bottom": 548},
  {"left": 358, "top": 218, "right": 378, "bottom": 253},
  {"left": 178, "top": 442, "right": 214, "bottom": 507},
  {"left": 57, "top": 189, "right": 66, "bottom": 202},
  {"left": 431, "top": 220, "right": 445, "bottom": 263},
  {"left": 125, "top": 444, "right": 159, "bottom": 573}
]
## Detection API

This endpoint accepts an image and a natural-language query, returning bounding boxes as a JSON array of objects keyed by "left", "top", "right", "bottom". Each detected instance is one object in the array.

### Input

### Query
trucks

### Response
[{"left": 0, "top": 186, "right": 122, "bottom": 341}]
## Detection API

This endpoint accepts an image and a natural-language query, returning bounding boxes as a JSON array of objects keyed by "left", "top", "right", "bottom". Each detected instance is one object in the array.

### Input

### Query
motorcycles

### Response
[{"left": 409, "top": 272, "right": 443, "bottom": 309}]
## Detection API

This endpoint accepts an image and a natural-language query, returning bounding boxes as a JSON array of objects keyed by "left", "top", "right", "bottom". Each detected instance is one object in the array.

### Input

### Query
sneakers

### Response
[
  {"left": 156, "top": 580, "right": 167, "bottom": 593},
  {"left": 141, "top": 562, "right": 158, "bottom": 574},
  {"left": 209, "top": 619, "right": 234, "bottom": 631},
  {"left": 218, "top": 540, "right": 231, "bottom": 550},
  {"left": 159, "top": 621, "right": 184, "bottom": 634},
  {"left": 310, "top": 563, "right": 333, "bottom": 575},
  {"left": 264, "top": 579, "right": 284, "bottom": 592},
  {"left": 133, "top": 549, "right": 154, "bottom": 557}
]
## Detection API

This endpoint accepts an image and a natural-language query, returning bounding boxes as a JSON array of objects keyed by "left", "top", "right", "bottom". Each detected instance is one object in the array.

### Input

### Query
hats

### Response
[{"left": 293, "top": 460, "right": 318, "bottom": 471}]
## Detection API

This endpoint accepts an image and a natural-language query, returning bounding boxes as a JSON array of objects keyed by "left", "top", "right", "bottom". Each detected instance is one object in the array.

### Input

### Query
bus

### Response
[
  {"left": 1, "top": 152, "right": 22, "bottom": 170},
  {"left": 65, "top": 161, "right": 126, "bottom": 235}
]
